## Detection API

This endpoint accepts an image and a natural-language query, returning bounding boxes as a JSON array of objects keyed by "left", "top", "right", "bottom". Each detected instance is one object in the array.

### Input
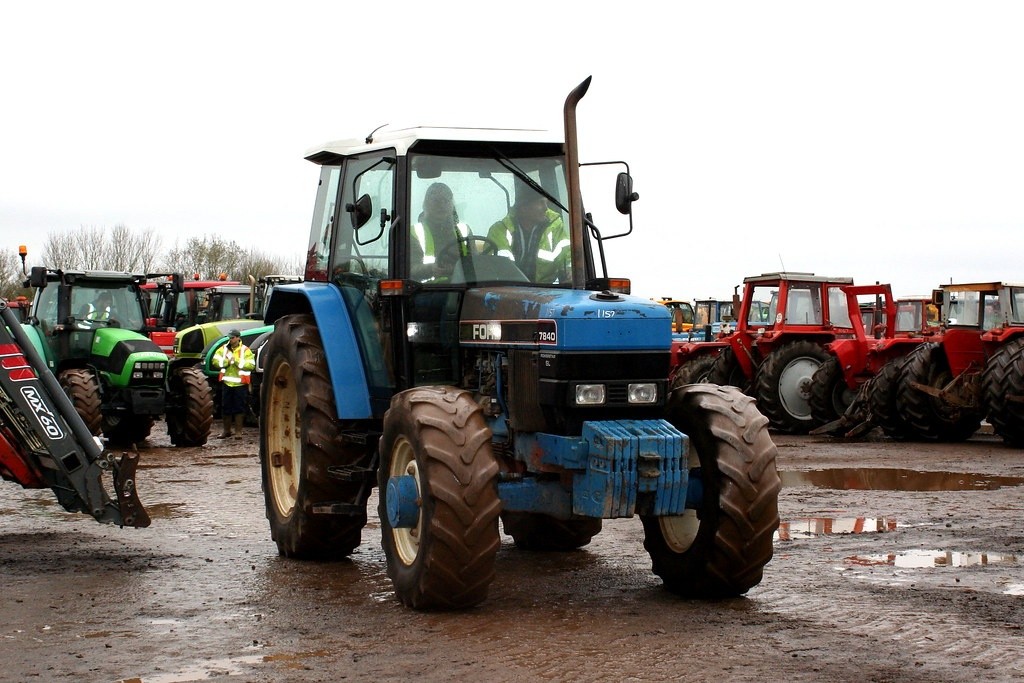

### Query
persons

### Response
[
  {"left": 77, "top": 292, "right": 113, "bottom": 330},
  {"left": 211, "top": 329, "right": 256, "bottom": 440},
  {"left": 481, "top": 188, "right": 574, "bottom": 286},
  {"left": 410, "top": 181, "right": 475, "bottom": 285},
  {"left": 202, "top": 293, "right": 260, "bottom": 320}
]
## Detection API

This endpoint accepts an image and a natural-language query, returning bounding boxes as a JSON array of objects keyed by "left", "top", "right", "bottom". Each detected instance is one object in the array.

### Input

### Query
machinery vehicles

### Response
[
  {"left": 0, "top": 297, "right": 152, "bottom": 530},
  {"left": 16, "top": 245, "right": 215, "bottom": 449},
  {"left": 235, "top": 74, "right": 784, "bottom": 613},
  {"left": 646, "top": 270, "right": 1023, "bottom": 446},
  {"left": 138, "top": 265, "right": 303, "bottom": 418}
]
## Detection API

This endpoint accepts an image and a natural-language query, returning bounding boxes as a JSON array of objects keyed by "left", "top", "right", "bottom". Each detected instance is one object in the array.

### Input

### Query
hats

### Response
[
  {"left": 227, "top": 328, "right": 240, "bottom": 337},
  {"left": 514, "top": 174, "right": 546, "bottom": 206}
]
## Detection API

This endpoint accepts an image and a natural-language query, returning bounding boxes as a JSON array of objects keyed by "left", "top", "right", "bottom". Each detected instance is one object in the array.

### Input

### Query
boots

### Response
[
  {"left": 217, "top": 415, "right": 231, "bottom": 438},
  {"left": 235, "top": 414, "right": 243, "bottom": 439}
]
